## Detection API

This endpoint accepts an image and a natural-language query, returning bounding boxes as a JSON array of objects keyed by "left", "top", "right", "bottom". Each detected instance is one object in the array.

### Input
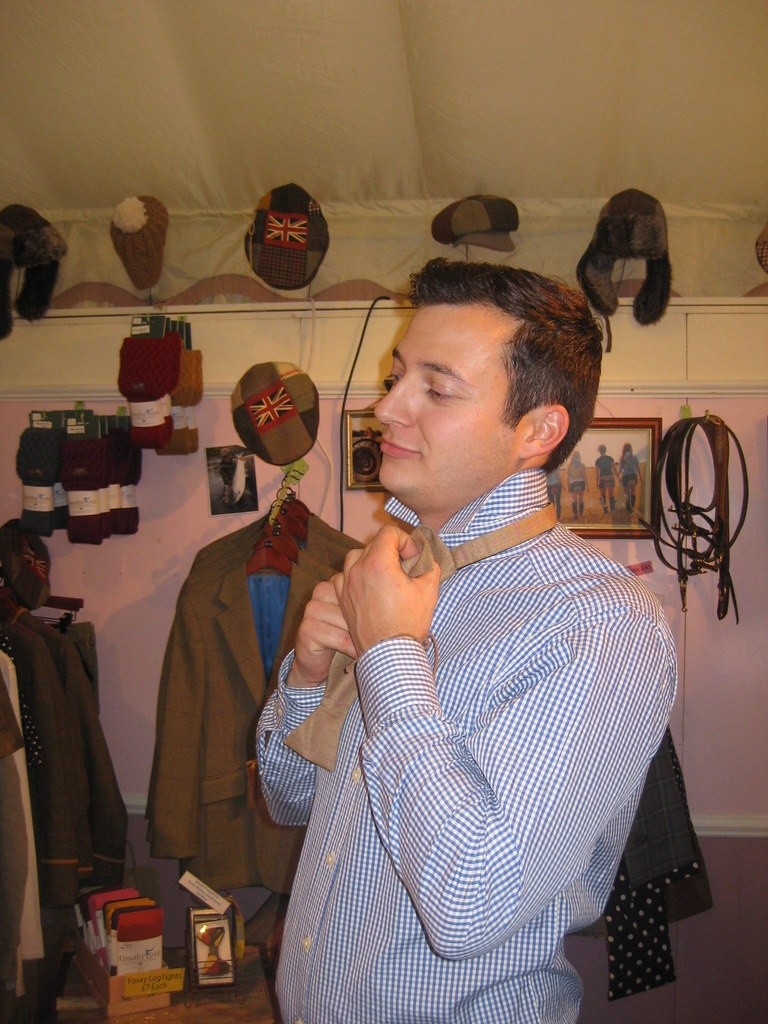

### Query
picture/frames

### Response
[
  {"left": 549, "top": 416, "right": 662, "bottom": 542},
  {"left": 344, "top": 408, "right": 385, "bottom": 491}
]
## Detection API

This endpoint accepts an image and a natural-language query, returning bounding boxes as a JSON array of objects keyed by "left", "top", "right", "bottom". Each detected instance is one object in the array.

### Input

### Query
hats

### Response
[
  {"left": 432, "top": 195, "right": 520, "bottom": 251},
  {"left": 232, "top": 360, "right": 321, "bottom": 465},
  {"left": 577, "top": 188, "right": 672, "bottom": 324},
  {"left": 110, "top": 194, "right": 168, "bottom": 286},
  {"left": 245, "top": 182, "right": 328, "bottom": 290},
  {"left": 0, "top": 518, "right": 53, "bottom": 609},
  {"left": 0, "top": 203, "right": 66, "bottom": 336}
]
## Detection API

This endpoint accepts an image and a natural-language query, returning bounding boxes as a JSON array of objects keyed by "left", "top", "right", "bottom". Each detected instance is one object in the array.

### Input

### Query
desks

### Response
[{"left": 60, "top": 942, "right": 277, "bottom": 1023}]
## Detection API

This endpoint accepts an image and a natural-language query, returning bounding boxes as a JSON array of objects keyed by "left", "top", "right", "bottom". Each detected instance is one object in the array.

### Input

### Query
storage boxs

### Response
[{"left": 74, "top": 943, "right": 170, "bottom": 1016}]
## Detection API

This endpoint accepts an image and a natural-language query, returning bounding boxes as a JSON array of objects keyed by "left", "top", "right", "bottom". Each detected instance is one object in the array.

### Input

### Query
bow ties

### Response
[{"left": 283, "top": 500, "right": 571, "bottom": 774}]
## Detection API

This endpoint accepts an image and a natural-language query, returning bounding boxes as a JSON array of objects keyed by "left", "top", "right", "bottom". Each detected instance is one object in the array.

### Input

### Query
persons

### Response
[
  {"left": 251, "top": 255, "right": 681, "bottom": 1022},
  {"left": 219, "top": 448, "right": 237, "bottom": 503},
  {"left": 619, "top": 442, "right": 642, "bottom": 513},
  {"left": 566, "top": 451, "right": 589, "bottom": 519},
  {"left": 546, "top": 466, "right": 565, "bottom": 521},
  {"left": 595, "top": 444, "right": 622, "bottom": 514}
]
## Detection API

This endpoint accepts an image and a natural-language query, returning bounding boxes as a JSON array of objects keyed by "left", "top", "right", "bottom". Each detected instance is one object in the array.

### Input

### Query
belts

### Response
[{"left": 637, "top": 412, "right": 749, "bottom": 623}]
